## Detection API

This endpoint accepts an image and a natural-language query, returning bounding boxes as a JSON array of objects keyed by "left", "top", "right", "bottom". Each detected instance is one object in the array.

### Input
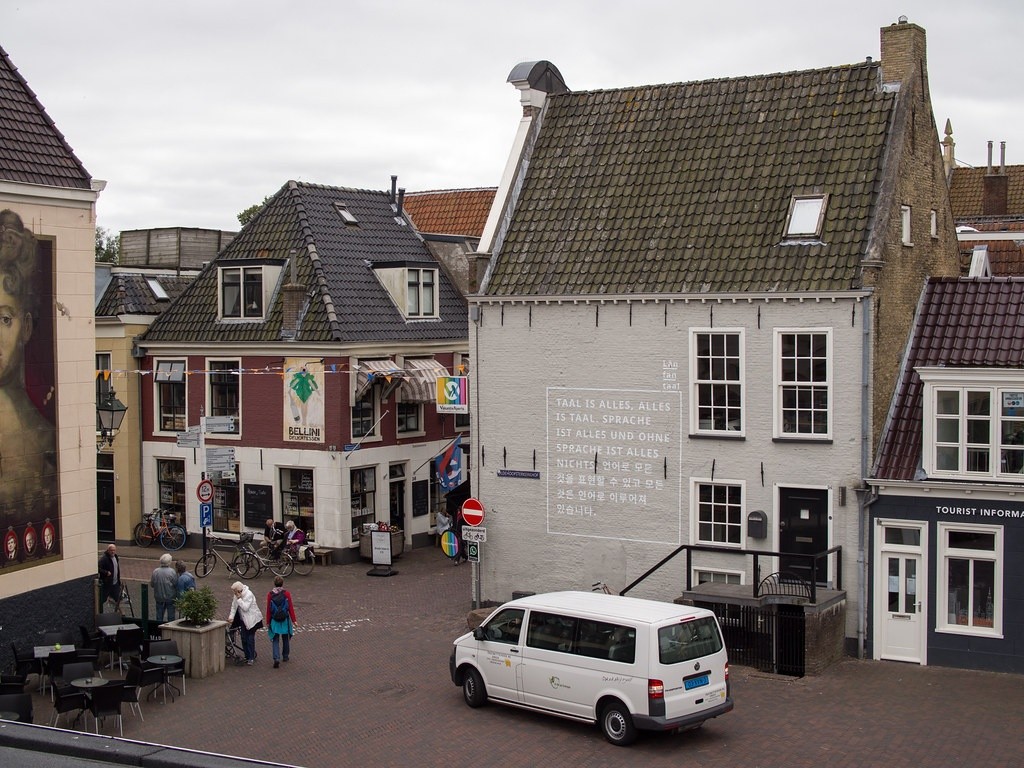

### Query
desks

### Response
[
  {"left": 147, "top": 655, "right": 183, "bottom": 703},
  {"left": 71, "top": 677, "right": 109, "bottom": 730},
  {"left": 33, "top": 644, "right": 76, "bottom": 696},
  {"left": 0, "top": 711, "right": 20, "bottom": 721},
  {"left": 99, "top": 623, "right": 144, "bottom": 671}
]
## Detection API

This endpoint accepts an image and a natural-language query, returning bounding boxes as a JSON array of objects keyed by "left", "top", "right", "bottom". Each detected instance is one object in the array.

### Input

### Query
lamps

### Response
[{"left": 96, "top": 386, "right": 128, "bottom": 447}]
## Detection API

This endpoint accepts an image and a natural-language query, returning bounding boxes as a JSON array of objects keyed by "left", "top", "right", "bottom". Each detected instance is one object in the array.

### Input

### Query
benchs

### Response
[{"left": 314, "top": 548, "right": 334, "bottom": 567}]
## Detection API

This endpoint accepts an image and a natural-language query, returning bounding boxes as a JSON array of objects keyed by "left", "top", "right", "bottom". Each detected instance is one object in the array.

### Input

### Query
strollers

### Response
[{"left": 223, "top": 612, "right": 257, "bottom": 665}]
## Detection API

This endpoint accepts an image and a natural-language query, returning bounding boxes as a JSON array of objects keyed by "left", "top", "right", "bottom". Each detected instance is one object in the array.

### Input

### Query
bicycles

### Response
[
  {"left": 194, "top": 529, "right": 315, "bottom": 579},
  {"left": 132, "top": 508, "right": 187, "bottom": 552}
]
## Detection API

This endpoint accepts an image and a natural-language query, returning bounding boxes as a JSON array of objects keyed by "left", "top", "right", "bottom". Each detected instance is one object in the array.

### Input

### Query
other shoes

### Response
[
  {"left": 273, "top": 661, "right": 280, "bottom": 668},
  {"left": 283, "top": 657, "right": 289, "bottom": 661},
  {"left": 454, "top": 560, "right": 459, "bottom": 565},
  {"left": 248, "top": 659, "right": 255, "bottom": 665}
]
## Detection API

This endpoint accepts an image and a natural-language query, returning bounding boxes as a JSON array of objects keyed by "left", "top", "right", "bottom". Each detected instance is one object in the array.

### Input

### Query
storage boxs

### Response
[{"left": 228, "top": 520, "right": 240, "bottom": 532}]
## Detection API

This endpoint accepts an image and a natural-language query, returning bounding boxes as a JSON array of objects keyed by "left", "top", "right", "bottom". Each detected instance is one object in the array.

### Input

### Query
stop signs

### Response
[{"left": 462, "top": 497, "right": 486, "bottom": 530}]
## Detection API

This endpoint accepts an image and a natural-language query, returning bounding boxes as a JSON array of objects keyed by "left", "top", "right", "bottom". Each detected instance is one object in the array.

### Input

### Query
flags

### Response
[{"left": 435, "top": 435, "right": 467, "bottom": 498}]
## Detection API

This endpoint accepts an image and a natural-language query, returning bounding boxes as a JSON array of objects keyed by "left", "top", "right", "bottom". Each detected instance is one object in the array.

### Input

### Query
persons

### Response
[
  {"left": 454, "top": 506, "right": 468, "bottom": 564},
  {"left": 286, "top": 520, "right": 309, "bottom": 560},
  {"left": 175, "top": 561, "right": 196, "bottom": 601},
  {"left": 26, "top": 531, "right": 36, "bottom": 554},
  {"left": 44, "top": 528, "right": 53, "bottom": 549},
  {"left": 436, "top": 507, "right": 453, "bottom": 535},
  {"left": 264, "top": 519, "right": 287, "bottom": 558},
  {"left": 228, "top": 581, "right": 264, "bottom": 666},
  {"left": 151, "top": 553, "right": 176, "bottom": 621},
  {"left": 6, "top": 536, "right": 16, "bottom": 558},
  {"left": 266, "top": 576, "right": 299, "bottom": 668},
  {"left": 99, "top": 544, "right": 122, "bottom": 613}
]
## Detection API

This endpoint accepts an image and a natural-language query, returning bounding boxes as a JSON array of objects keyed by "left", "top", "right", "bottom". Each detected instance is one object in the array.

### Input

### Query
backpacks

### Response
[{"left": 270, "top": 590, "right": 290, "bottom": 622}]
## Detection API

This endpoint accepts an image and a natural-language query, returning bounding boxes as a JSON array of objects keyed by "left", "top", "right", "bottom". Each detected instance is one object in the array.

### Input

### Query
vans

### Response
[{"left": 446, "top": 589, "right": 733, "bottom": 746}]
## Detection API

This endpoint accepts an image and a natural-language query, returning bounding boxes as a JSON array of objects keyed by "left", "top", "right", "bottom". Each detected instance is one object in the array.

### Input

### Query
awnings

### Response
[
  {"left": 354, "top": 360, "right": 406, "bottom": 403},
  {"left": 402, "top": 358, "right": 451, "bottom": 403}
]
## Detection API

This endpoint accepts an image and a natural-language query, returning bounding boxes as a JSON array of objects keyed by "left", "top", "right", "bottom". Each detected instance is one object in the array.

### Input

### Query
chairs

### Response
[
  {"left": 129, "top": 656, "right": 167, "bottom": 704},
  {"left": 139, "top": 638, "right": 171, "bottom": 661},
  {"left": 1, "top": 674, "right": 23, "bottom": 684},
  {"left": 10, "top": 642, "right": 43, "bottom": 677},
  {"left": 46, "top": 632, "right": 74, "bottom": 646},
  {"left": 109, "top": 665, "right": 144, "bottom": 729},
  {"left": 44, "top": 651, "right": 77, "bottom": 703},
  {"left": 84, "top": 683, "right": 124, "bottom": 737},
  {"left": 0, "top": 694, "right": 34, "bottom": 724},
  {"left": 111, "top": 626, "right": 143, "bottom": 677},
  {"left": 63, "top": 662, "right": 95, "bottom": 684},
  {"left": 78, "top": 624, "right": 112, "bottom": 666},
  {"left": 149, "top": 641, "right": 186, "bottom": 699},
  {"left": 95, "top": 615, "right": 122, "bottom": 632},
  {"left": 76, "top": 637, "right": 103, "bottom": 672},
  {"left": 49, "top": 674, "right": 85, "bottom": 728},
  {"left": 0, "top": 683, "right": 24, "bottom": 695}
]
